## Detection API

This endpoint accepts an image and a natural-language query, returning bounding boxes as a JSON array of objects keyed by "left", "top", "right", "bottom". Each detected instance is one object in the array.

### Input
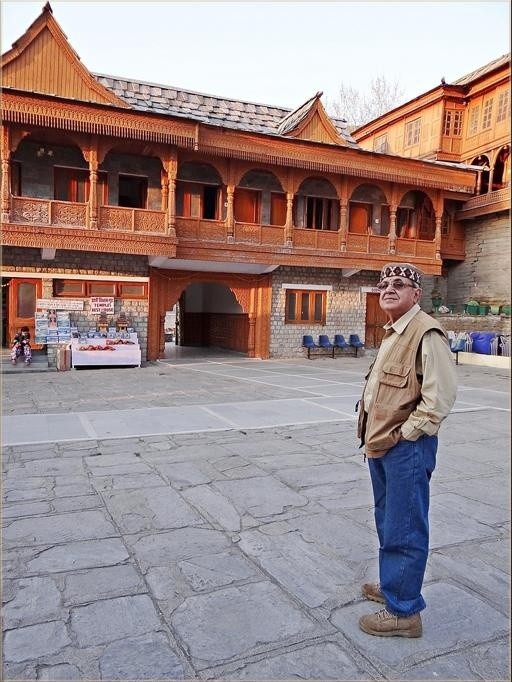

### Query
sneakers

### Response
[
  {"left": 12, "top": 362, "right": 30, "bottom": 366},
  {"left": 361, "top": 583, "right": 389, "bottom": 605},
  {"left": 358, "top": 608, "right": 423, "bottom": 638}
]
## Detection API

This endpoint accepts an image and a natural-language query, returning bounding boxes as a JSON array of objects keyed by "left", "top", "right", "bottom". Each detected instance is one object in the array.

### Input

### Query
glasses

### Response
[{"left": 377, "top": 281, "right": 416, "bottom": 290}]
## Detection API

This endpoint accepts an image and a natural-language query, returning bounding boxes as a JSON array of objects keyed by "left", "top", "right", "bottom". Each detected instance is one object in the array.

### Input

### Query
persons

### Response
[
  {"left": 356, "top": 263, "right": 457, "bottom": 638},
  {"left": 10, "top": 326, "right": 32, "bottom": 366}
]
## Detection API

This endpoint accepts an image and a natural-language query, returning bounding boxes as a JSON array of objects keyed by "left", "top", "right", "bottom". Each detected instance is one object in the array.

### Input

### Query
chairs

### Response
[{"left": 302, "top": 335, "right": 364, "bottom": 359}]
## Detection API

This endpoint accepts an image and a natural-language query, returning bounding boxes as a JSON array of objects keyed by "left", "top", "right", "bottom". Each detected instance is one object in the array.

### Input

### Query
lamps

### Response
[{"left": 36, "top": 142, "right": 54, "bottom": 158}]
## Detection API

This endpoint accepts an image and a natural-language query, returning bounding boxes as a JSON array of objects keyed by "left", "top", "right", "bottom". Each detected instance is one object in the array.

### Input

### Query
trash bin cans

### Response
[{"left": 56, "top": 349, "right": 71, "bottom": 371}]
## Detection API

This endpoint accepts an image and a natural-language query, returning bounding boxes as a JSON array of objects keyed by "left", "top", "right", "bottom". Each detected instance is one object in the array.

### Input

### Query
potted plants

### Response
[{"left": 467, "top": 301, "right": 479, "bottom": 316}]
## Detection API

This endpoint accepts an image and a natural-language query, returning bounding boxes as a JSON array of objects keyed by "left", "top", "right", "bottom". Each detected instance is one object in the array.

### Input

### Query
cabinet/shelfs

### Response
[{"left": 71, "top": 332, "right": 141, "bottom": 368}]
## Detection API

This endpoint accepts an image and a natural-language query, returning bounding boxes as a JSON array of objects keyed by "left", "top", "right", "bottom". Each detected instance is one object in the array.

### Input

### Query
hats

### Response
[{"left": 379, "top": 262, "right": 426, "bottom": 283}]
